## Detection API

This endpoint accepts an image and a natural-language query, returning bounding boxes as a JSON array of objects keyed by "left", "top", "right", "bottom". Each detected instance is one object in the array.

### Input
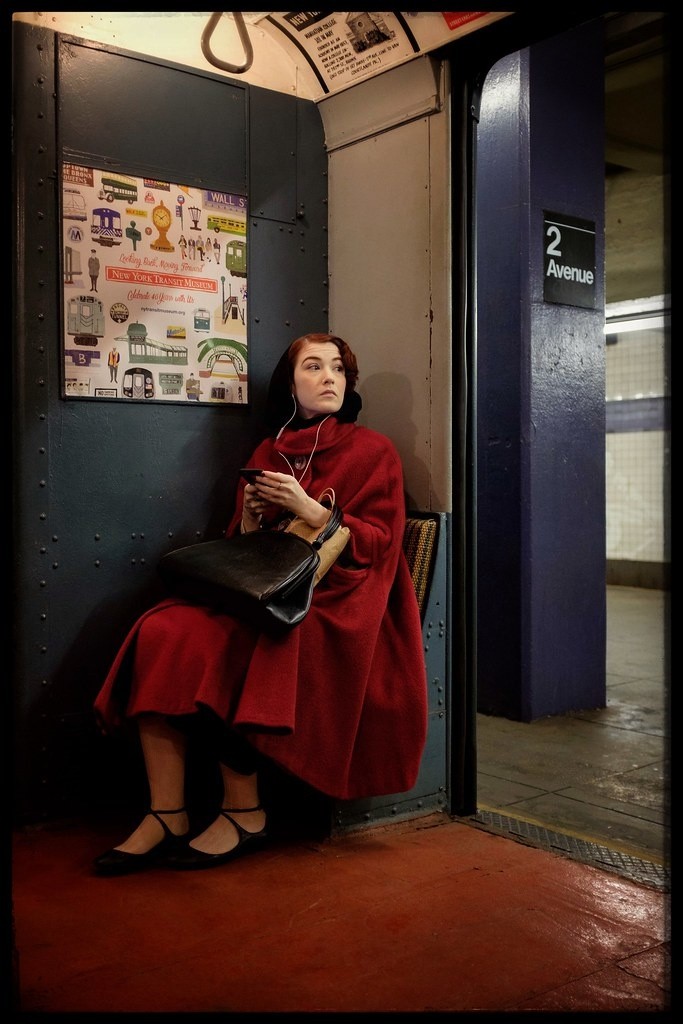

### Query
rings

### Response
[{"left": 277, "top": 482, "right": 282, "bottom": 490}]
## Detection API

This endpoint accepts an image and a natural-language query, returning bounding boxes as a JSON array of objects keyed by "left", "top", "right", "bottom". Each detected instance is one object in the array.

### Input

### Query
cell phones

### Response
[{"left": 240, "top": 469, "right": 263, "bottom": 486}]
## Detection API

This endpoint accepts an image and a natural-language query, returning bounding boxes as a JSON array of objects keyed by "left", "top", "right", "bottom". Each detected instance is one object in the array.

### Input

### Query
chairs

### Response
[{"left": 324, "top": 508, "right": 447, "bottom": 836}]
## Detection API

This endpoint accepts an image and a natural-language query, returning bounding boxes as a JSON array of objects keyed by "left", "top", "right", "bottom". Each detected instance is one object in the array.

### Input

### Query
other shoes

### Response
[
  {"left": 162, "top": 804, "right": 273, "bottom": 870},
  {"left": 92, "top": 801, "right": 195, "bottom": 875}
]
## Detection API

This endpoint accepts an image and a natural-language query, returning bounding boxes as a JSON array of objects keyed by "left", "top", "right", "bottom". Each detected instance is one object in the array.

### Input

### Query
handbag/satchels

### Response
[
  {"left": 284, "top": 488, "right": 350, "bottom": 588},
  {"left": 162, "top": 504, "right": 344, "bottom": 641}
]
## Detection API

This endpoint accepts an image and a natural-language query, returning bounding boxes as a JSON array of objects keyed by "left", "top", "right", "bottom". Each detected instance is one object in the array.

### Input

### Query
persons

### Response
[{"left": 95, "top": 333, "right": 429, "bottom": 882}]
